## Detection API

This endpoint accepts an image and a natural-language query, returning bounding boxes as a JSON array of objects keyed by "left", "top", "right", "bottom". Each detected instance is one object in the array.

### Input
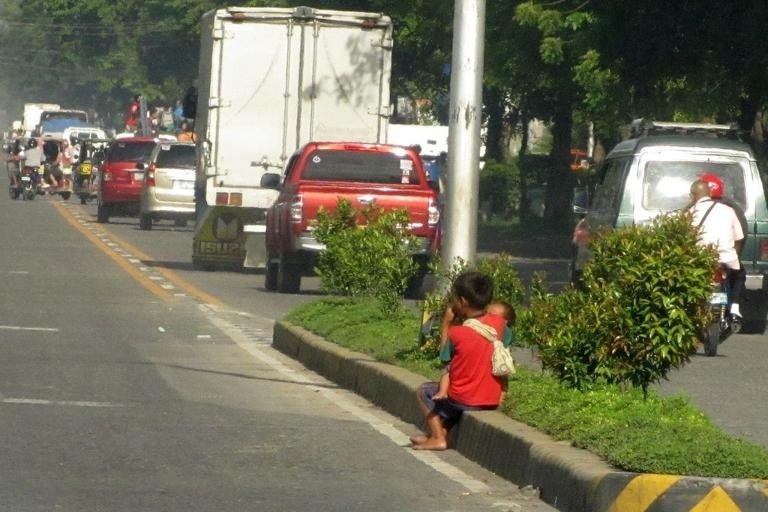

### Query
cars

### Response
[
  {"left": 137, "top": 141, "right": 200, "bottom": 230},
  {"left": 97, "top": 138, "right": 158, "bottom": 224}
]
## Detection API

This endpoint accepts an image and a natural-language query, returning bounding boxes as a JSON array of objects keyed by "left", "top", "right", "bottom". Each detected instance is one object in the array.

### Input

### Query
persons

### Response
[
  {"left": 429, "top": 303, "right": 515, "bottom": 402},
  {"left": 681, "top": 173, "right": 746, "bottom": 256},
  {"left": 409, "top": 272, "right": 515, "bottom": 454},
  {"left": 683, "top": 180, "right": 744, "bottom": 324},
  {"left": 0, "top": 135, "right": 110, "bottom": 203},
  {"left": 411, "top": 144, "right": 449, "bottom": 194}
]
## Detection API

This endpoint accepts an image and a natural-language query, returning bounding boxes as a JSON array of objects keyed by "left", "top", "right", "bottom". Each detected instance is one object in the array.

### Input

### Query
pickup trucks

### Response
[{"left": 261, "top": 142, "right": 439, "bottom": 299}]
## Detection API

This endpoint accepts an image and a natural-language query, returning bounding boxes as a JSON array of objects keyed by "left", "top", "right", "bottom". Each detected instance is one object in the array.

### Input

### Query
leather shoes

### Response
[{"left": 729, "top": 313, "right": 744, "bottom": 323}]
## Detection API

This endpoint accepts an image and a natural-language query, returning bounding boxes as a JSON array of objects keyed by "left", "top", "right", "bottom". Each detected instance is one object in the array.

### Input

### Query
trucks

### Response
[
  {"left": 5, "top": 103, "right": 109, "bottom": 151},
  {"left": 182, "top": 5, "right": 394, "bottom": 213}
]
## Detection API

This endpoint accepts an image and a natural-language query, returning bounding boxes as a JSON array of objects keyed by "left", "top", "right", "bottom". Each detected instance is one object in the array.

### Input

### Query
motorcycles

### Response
[
  {"left": 8, "top": 155, "right": 95, "bottom": 205},
  {"left": 686, "top": 262, "right": 743, "bottom": 358}
]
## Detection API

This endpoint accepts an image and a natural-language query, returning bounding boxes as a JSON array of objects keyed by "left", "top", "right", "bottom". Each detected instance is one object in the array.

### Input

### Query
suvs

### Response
[{"left": 569, "top": 116, "right": 768, "bottom": 332}]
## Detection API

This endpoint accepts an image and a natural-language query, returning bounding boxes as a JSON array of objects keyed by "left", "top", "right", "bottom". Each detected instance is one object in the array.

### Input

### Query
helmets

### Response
[{"left": 702, "top": 175, "right": 723, "bottom": 198}]
